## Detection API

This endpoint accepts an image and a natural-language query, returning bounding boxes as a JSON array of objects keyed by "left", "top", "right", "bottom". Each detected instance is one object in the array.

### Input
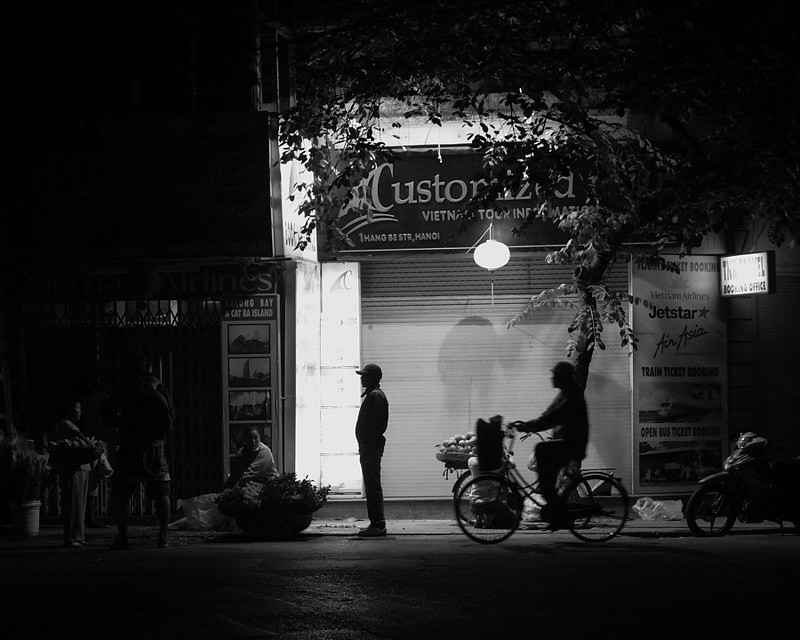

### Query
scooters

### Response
[{"left": 685, "top": 430, "right": 800, "bottom": 538}]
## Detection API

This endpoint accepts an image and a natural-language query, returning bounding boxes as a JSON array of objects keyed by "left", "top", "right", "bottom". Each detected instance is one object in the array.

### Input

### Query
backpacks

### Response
[{"left": 476, "top": 414, "right": 502, "bottom": 471}]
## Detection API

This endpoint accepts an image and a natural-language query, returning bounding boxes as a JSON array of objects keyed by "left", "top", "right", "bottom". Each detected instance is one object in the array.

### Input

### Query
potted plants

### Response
[{"left": 214, "top": 473, "right": 331, "bottom": 540}]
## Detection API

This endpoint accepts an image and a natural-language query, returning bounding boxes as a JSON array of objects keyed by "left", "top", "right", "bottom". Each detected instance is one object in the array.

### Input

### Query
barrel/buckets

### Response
[{"left": 13, "top": 501, "right": 41, "bottom": 537}]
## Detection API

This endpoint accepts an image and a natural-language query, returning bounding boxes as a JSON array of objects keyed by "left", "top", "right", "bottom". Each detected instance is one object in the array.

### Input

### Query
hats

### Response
[
  {"left": 356, "top": 363, "right": 382, "bottom": 375},
  {"left": 547, "top": 362, "right": 576, "bottom": 374}
]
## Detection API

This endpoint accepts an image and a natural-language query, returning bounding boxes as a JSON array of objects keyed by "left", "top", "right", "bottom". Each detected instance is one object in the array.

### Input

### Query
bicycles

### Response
[{"left": 450, "top": 420, "right": 635, "bottom": 544}]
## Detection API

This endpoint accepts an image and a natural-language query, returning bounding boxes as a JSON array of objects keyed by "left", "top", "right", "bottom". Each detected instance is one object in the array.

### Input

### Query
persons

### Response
[
  {"left": 355, "top": 364, "right": 389, "bottom": 536},
  {"left": 56, "top": 396, "right": 105, "bottom": 547},
  {"left": 216, "top": 425, "right": 280, "bottom": 489},
  {"left": 107, "top": 349, "right": 174, "bottom": 550},
  {"left": 516, "top": 361, "right": 589, "bottom": 532}
]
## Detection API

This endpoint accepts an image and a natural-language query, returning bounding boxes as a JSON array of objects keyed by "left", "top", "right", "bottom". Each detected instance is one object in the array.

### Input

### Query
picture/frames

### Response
[
  {"left": 228, "top": 424, "right": 277, "bottom": 472},
  {"left": 226, "top": 322, "right": 271, "bottom": 355},
  {"left": 228, "top": 390, "right": 272, "bottom": 421},
  {"left": 228, "top": 356, "right": 271, "bottom": 388}
]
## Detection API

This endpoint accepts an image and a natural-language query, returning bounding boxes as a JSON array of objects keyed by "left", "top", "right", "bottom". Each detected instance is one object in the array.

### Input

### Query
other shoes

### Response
[
  {"left": 110, "top": 537, "right": 129, "bottom": 550},
  {"left": 64, "top": 540, "right": 88, "bottom": 549},
  {"left": 359, "top": 525, "right": 388, "bottom": 536},
  {"left": 157, "top": 536, "right": 170, "bottom": 548}
]
